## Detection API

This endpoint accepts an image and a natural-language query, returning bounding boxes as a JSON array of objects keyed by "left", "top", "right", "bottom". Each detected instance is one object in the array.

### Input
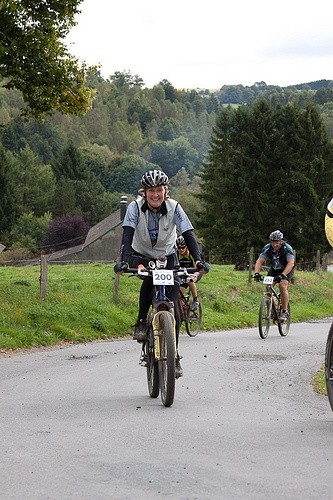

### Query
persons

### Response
[
  {"left": 254, "top": 230, "right": 296, "bottom": 320},
  {"left": 324, "top": 198, "right": 333, "bottom": 247},
  {"left": 177, "top": 236, "right": 203, "bottom": 310},
  {"left": 113, "top": 169, "right": 209, "bottom": 379}
]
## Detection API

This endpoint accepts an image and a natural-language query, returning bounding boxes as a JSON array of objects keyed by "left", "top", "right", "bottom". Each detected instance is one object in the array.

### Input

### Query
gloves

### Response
[
  {"left": 254, "top": 272, "right": 263, "bottom": 282},
  {"left": 273, "top": 273, "right": 287, "bottom": 283}
]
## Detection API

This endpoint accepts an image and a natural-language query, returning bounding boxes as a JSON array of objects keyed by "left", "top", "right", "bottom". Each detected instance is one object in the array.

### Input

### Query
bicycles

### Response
[
  {"left": 173, "top": 276, "right": 203, "bottom": 337},
  {"left": 252, "top": 274, "right": 292, "bottom": 340},
  {"left": 114, "top": 266, "right": 201, "bottom": 409}
]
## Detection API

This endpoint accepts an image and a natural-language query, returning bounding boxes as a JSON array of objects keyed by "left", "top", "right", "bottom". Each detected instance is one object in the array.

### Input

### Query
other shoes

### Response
[
  {"left": 176, "top": 354, "right": 184, "bottom": 377},
  {"left": 190, "top": 301, "right": 199, "bottom": 310},
  {"left": 131, "top": 318, "right": 147, "bottom": 340},
  {"left": 279, "top": 309, "right": 289, "bottom": 320}
]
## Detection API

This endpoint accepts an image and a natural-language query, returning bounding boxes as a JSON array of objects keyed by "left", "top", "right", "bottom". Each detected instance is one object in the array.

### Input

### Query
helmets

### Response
[
  {"left": 176, "top": 236, "right": 185, "bottom": 246},
  {"left": 140, "top": 170, "right": 169, "bottom": 189},
  {"left": 269, "top": 230, "right": 283, "bottom": 241}
]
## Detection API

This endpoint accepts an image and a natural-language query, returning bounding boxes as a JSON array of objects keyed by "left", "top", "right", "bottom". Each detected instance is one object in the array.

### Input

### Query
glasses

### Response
[{"left": 177, "top": 246, "right": 186, "bottom": 249}]
